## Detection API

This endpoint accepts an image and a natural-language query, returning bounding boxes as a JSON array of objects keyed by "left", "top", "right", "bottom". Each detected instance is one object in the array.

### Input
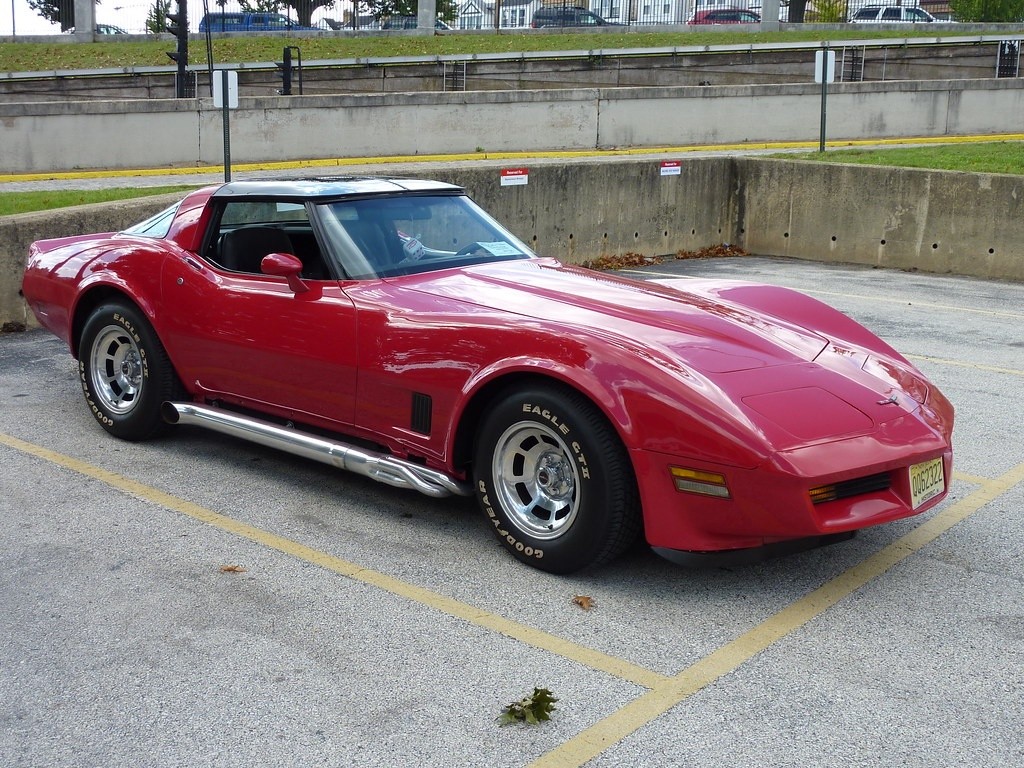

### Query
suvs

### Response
[
  {"left": 531, "top": 6, "right": 630, "bottom": 25},
  {"left": 849, "top": 3, "right": 959, "bottom": 24},
  {"left": 685, "top": 9, "right": 785, "bottom": 23}
]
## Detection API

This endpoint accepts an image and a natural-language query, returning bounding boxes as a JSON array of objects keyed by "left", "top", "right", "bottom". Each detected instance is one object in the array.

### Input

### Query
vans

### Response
[{"left": 382, "top": 16, "right": 455, "bottom": 31}]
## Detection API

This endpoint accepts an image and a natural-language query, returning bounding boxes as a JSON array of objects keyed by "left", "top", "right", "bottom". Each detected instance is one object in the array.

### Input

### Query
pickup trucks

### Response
[{"left": 198, "top": 12, "right": 323, "bottom": 30}]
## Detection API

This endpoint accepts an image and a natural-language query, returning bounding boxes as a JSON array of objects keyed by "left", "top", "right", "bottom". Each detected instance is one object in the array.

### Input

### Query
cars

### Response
[{"left": 71, "top": 24, "right": 129, "bottom": 35}]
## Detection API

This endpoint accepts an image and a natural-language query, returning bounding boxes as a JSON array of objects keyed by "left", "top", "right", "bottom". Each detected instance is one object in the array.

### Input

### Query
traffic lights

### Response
[
  {"left": 274, "top": 47, "right": 292, "bottom": 95},
  {"left": 166, "top": 0, "right": 188, "bottom": 66}
]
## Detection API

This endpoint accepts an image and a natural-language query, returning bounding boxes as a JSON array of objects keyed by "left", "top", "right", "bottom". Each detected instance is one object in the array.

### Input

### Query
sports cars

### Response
[{"left": 21, "top": 172, "right": 958, "bottom": 575}]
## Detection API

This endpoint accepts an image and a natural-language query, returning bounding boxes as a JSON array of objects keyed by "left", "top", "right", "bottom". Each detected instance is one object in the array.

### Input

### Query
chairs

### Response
[
  {"left": 340, "top": 220, "right": 396, "bottom": 273},
  {"left": 219, "top": 224, "right": 296, "bottom": 274}
]
